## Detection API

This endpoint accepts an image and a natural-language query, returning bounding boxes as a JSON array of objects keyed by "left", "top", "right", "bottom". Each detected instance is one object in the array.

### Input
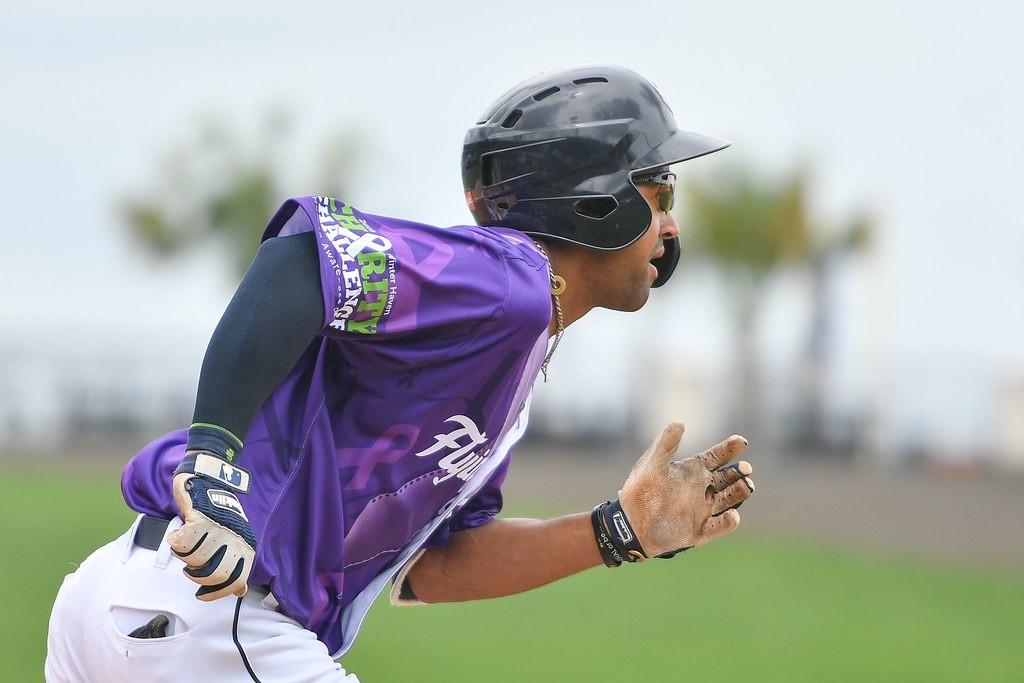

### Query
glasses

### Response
[{"left": 630, "top": 171, "right": 676, "bottom": 212}]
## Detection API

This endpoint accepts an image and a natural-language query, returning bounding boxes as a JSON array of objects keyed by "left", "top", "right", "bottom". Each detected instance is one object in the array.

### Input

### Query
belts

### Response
[{"left": 133, "top": 516, "right": 285, "bottom": 615}]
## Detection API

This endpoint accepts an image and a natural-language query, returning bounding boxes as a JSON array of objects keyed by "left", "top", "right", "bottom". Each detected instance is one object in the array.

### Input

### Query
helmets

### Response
[{"left": 461, "top": 66, "right": 732, "bottom": 288}]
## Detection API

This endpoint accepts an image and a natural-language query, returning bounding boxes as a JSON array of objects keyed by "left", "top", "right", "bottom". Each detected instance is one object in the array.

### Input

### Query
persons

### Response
[{"left": 46, "top": 65, "right": 754, "bottom": 682}]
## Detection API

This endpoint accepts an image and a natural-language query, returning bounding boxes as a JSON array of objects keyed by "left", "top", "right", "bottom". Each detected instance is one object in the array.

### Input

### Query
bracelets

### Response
[{"left": 592, "top": 498, "right": 647, "bottom": 569}]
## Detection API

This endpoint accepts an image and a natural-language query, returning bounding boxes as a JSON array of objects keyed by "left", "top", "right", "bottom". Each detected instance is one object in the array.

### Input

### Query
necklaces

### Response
[{"left": 531, "top": 236, "right": 564, "bottom": 384}]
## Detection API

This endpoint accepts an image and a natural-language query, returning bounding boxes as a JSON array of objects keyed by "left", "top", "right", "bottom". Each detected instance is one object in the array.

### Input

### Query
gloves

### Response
[
  {"left": 588, "top": 421, "right": 755, "bottom": 569},
  {"left": 169, "top": 450, "right": 252, "bottom": 602}
]
